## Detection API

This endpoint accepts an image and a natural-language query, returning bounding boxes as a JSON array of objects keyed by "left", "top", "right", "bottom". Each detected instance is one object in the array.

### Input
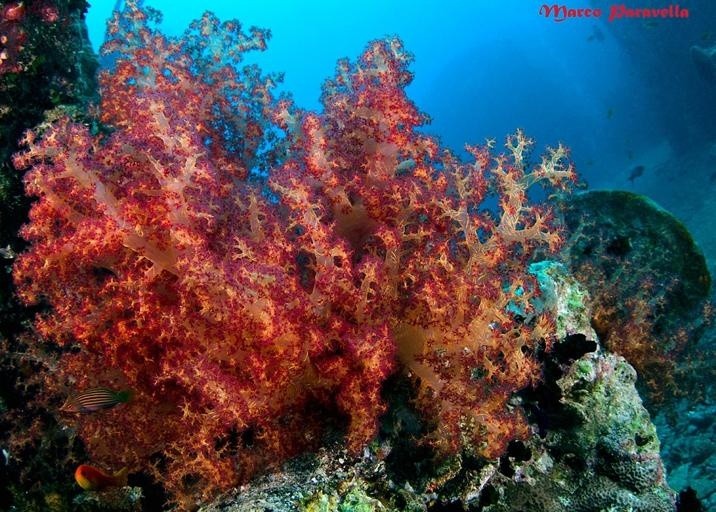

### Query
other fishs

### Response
[
  {"left": 58, "top": 385, "right": 132, "bottom": 414},
  {"left": 74, "top": 463, "right": 128, "bottom": 493}
]
